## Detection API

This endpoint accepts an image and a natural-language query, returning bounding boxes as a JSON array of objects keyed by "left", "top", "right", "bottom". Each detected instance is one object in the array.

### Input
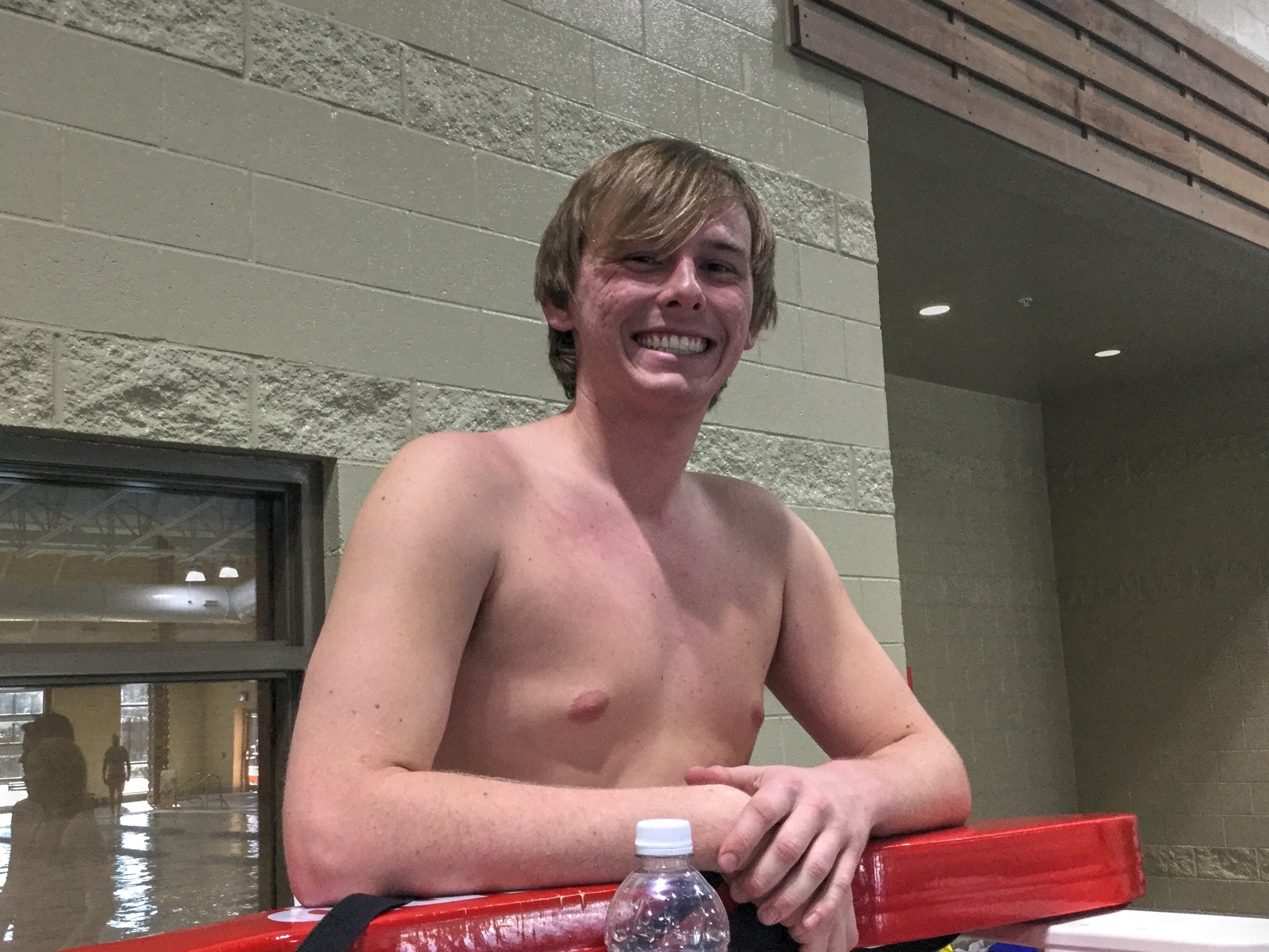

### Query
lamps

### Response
[{"left": 184, "top": 537, "right": 239, "bottom": 582}]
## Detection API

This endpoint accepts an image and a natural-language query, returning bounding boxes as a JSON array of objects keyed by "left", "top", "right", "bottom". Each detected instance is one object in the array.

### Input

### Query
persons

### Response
[
  {"left": 0, "top": 710, "right": 120, "bottom": 951},
  {"left": 101, "top": 732, "right": 132, "bottom": 817},
  {"left": 277, "top": 135, "right": 1049, "bottom": 951}
]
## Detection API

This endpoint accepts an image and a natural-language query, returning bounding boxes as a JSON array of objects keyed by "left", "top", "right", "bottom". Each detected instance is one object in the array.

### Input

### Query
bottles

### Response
[{"left": 601, "top": 816, "right": 732, "bottom": 951}]
